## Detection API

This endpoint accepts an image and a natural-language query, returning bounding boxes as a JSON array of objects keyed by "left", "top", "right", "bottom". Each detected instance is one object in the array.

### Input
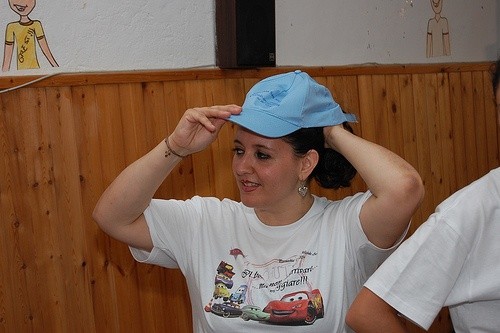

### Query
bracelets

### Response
[{"left": 165, "top": 137, "right": 190, "bottom": 160}]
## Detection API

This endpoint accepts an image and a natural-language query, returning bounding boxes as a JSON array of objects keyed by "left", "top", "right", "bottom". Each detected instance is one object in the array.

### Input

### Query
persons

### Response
[
  {"left": 345, "top": 58, "right": 500, "bottom": 333},
  {"left": 92, "top": 70, "right": 424, "bottom": 333}
]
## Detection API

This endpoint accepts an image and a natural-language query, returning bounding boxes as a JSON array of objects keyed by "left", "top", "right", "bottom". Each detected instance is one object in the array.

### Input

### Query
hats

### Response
[{"left": 224, "top": 70, "right": 358, "bottom": 137}]
